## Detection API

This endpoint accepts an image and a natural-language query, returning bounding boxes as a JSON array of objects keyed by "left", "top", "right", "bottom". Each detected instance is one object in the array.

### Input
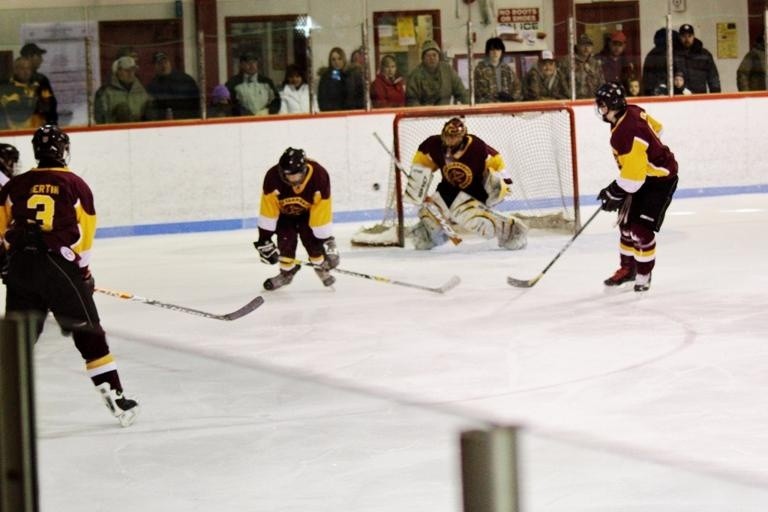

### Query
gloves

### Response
[
  {"left": 253, "top": 238, "right": 280, "bottom": 265},
  {"left": 596, "top": 181, "right": 627, "bottom": 211},
  {"left": 320, "top": 238, "right": 340, "bottom": 271}
]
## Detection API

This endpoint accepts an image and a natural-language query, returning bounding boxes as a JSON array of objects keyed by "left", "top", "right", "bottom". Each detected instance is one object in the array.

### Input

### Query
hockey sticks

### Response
[
  {"left": 372, "top": 132, "right": 491, "bottom": 252},
  {"left": 278, "top": 255, "right": 460, "bottom": 294},
  {"left": 508, "top": 203, "right": 602, "bottom": 287},
  {"left": 94, "top": 288, "right": 264, "bottom": 320}
]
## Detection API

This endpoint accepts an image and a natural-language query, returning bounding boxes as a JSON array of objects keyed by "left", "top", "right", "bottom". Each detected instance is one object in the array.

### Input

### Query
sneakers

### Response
[
  {"left": 263, "top": 270, "right": 293, "bottom": 290},
  {"left": 635, "top": 270, "right": 651, "bottom": 291},
  {"left": 115, "top": 398, "right": 136, "bottom": 409},
  {"left": 604, "top": 264, "right": 635, "bottom": 286},
  {"left": 311, "top": 263, "right": 336, "bottom": 286}
]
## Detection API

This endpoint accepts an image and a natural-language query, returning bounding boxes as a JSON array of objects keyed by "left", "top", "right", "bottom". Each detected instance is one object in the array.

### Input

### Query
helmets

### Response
[
  {"left": 0, "top": 143, "right": 19, "bottom": 176},
  {"left": 32, "top": 124, "right": 70, "bottom": 167},
  {"left": 440, "top": 118, "right": 467, "bottom": 157},
  {"left": 278, "top": 147, "right": 308, "bottom": 186},
  {"left": 594, "top": 82, "right": 626, "bottom": 110}
]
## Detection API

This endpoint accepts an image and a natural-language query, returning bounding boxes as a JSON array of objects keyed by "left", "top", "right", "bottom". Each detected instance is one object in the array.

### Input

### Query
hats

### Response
[
  {"left": 212, "top": 85, "right": 231, "bottom": 97},
  {"left": 577, "top": 34, "right": 594, "bottom": 45},
  {"left": 421, "top": 40, "right": 440, "bottom": 57},
  {"left": 239, "top": 51, "right": 257, "bottom": 62},
  {"left": 151, "top": 51, "right": 167, "bottom": 62},
  {"left": 21, "top": 44, "right": 46, "bottom": 57},
  {"left": 118, "top": 56, "right": 139, "bottom": 70},
  {"left": 610, "top": 31, "right": 626, "bottom": 43},
  {"left": 538, "top": 50, "right": 554, "bottom": 62},
  {"left": 679, "top": 24, "right": 693, "bottom": 34}
]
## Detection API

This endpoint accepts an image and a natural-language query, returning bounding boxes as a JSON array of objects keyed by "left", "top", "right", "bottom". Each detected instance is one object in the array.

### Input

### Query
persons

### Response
[
  {"left": 1, "top": 44, "right": 58, "bottom": 131},
  {"left": 401, "top": 117, "right": 530, "bottom": 252},
  {"left": 593, "top": 80, "right": 680, "bottom": 300},
  {"left": 0, "top": 143, "right": 20, "bottom": 285},
  {"left": 206, "top": 43, "right": 368, "bottom": 119},
  {"left": 737, "top": 29, "right": 768, "bottom": 91},
  {"left": 0, "top": 124, "right": 141, "bottom": 430},
  {"left": 370, "top": 36, "right": 571, "bottom": 109},
  {"left": 94, "top": 47, "right": 203, "bottom": 124},
  {"left": 252, "top": 146, "right": 340, "bottom": 295},
  {"left": 640, "top": 24, "right": 720, "bottom": 96},
  {"left": 558, "top": 30, "right": 640, "bottom": 100}
]
